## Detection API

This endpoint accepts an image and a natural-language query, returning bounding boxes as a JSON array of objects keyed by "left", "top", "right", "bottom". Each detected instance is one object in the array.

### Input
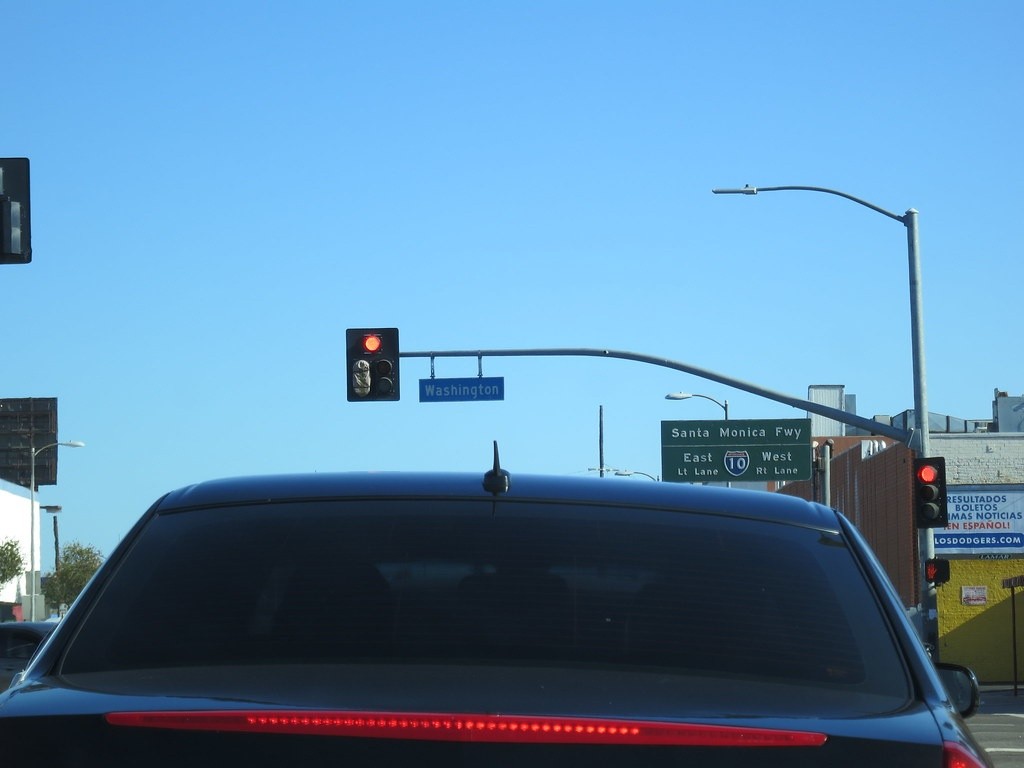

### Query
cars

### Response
[
  {"left": 0, "top": 620, "right": 64, "bottom": 692},
  {"left": 0, "top": 468, "right": 995, "bottom": 767}
]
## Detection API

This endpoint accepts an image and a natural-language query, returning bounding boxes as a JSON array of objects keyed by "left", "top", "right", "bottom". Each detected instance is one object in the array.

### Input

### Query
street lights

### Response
[
  {"left": 30, "top": 440, "right": 88, "bottom": 622},
  {"left": 663, "top": 393, "right": 735, "bottom": 487},
  {"left": 710, "top": 183, "right": 942, "bottom": 672}
]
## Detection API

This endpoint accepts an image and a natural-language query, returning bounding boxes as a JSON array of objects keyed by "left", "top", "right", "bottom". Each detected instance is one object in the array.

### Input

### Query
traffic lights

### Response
[
  {"left": 924, "top": 555, "right": 951, "bottom": 585},
  {"left": 373, "top": 357, "right": 395, "bottom": 398},
  {"left": 914, "top": 456, "right": 948, "bottom": 529},
  {"left": 361, "top": 332, "right": 385, "bottom": 354}
]
